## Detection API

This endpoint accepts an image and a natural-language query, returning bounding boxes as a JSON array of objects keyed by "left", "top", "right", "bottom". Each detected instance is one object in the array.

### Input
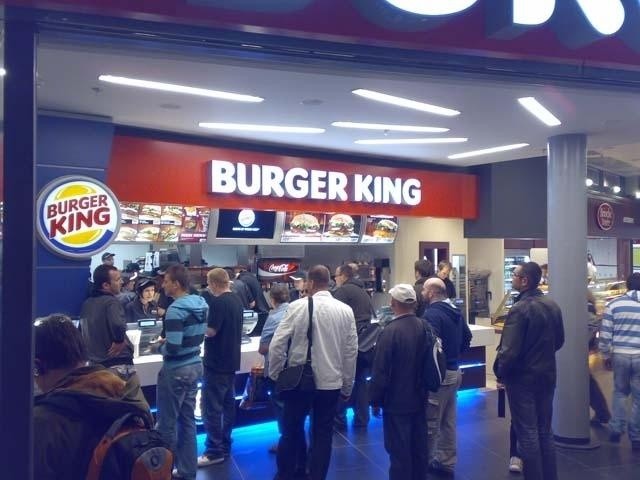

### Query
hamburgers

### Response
[
  {"left": 117, "top": 203, "right": 186, "bottom": 242},
  {"left": 290, "top": 214, "right": 320, "bottom": 234},
  {"left": 328, "top": 213, "right": 355, "bottom": 235},
  {"left": 373, "top": 219, "right": 398, "bottom": 238}
]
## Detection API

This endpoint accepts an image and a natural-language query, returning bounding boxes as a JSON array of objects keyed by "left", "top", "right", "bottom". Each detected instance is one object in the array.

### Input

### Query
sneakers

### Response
[
  {"left": 591, "top": 410, "right": 611, "bottom": 425},
  {"left": 632, "top": 439, "right": 639, "bottom": 452},
  {"left": 508, "top": 456, "right": 525, "bottom": 472},
  {"left": 196, "top": 454, "right": 225, "bottom": 466},
  {"left": 607, "top": 432, "right": 622, "bottom": 443}
]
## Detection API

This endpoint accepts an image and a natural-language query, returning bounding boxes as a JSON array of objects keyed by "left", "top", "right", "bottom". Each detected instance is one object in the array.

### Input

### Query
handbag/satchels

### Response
[
  {"left": 356, "top": 322, "right": 384, "bottom": 362},
  {"left": 238, "top": 362, "right": 270, "bottom": 413},
  {"left": 275, "top": 364, "right": 316, "bottom": 403}
]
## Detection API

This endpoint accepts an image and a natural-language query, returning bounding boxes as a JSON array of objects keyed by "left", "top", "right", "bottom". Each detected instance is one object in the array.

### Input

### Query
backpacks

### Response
[
  {"left": 36, "top": 396, "right": 174, "bottom": 480},
  {"left": 417, "top": 317, "right": 447, "bottom": 393}
]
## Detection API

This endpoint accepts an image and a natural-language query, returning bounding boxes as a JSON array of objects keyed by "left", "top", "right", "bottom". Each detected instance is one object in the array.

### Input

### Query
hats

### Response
[
  {"left": 287, "top": 270, "right": 307, "bottom": 281},
  {"left": 135, "top": 278, "right": 157, "bottom": 290},
  {"left": 387, "top": 282, "right": 418, "bottom": 306},
  {"left": 101, "top": 251, "right": 116, "bottom": 260}
]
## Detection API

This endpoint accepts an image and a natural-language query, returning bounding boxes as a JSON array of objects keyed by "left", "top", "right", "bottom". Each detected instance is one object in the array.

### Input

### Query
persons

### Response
[
  {"left": 597, "top": 272, "right": 640, "bottom": 452},
  {"left": 33, "top": 314, "right": 155, "bottom": 479},
  {"left": 508, "top": 417, "right": 524, "bottom": 472},
  {"left": 587, "top": 260, "right": 611, "bottom": 424},
  {"left": 495, "top": 262, "right": 565, "bottom": 480},
  {"left": 79, "top": 252, "right": 472, "bottom": 478}
]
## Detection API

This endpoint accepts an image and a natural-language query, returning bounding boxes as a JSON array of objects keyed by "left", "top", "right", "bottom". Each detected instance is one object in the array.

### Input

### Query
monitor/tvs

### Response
[
  {"left": 241, "top": 313, "right": 258, "bottom": 344},
  {"left": 208, "top": 208, "right": 285, "bottom": 245},
  {"left": 126, "top": 321, "right": 164, "bottom": 355}
]
